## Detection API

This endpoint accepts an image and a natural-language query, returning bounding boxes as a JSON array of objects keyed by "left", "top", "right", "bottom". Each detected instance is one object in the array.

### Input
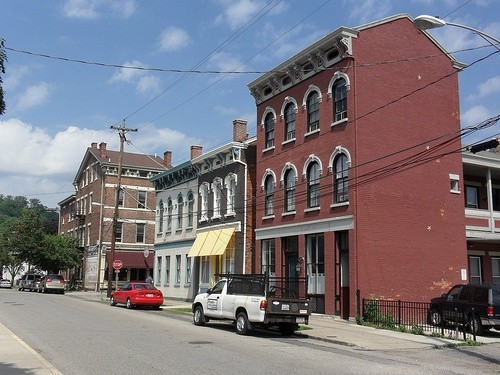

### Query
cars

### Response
[
  {"left": 109, "top": 282, "right": 164, "bottom": 309},
  {"left": 0, "top": 279, "right": 12, "bottom": 288}
]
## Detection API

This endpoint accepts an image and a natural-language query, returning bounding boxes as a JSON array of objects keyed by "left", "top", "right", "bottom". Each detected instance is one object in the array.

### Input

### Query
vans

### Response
[{"left": 18, "top": 274, "right": 42, "bottom": 292}]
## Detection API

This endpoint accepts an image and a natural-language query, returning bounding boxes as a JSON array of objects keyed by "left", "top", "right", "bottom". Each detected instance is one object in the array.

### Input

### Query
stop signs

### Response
[{"left": 112, "top": 259, "right": 123, "bottom": 269}]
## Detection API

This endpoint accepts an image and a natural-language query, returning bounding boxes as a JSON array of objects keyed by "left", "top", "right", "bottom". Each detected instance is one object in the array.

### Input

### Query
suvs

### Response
[
  {"left": 427, "top": 284, "right": 500, "bottom": 336},
  {"left": 39, "top": 274, "right": 65, "bottom": 294}
]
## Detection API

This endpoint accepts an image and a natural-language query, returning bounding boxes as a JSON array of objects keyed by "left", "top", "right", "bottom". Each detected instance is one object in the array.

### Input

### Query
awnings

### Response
[
  {"left": 186, "top": 228, "right": 237, "bottom": 258},
  {"left": 141, "top": 251, "right": 153, "bottom": 268},
  {"left": 106, "top": 251, "right": 146, "bottom": 268}
]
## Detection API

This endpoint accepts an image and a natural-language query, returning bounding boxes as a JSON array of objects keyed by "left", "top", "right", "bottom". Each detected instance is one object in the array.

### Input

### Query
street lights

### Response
[{"left": 234, "top": 159, "right": 247, "bottom": 274}]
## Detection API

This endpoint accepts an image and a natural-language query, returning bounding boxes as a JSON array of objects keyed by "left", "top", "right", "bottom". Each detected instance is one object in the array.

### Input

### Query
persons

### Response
[{"left": 144, "top": 272, "right": 153, "bottom": 284}]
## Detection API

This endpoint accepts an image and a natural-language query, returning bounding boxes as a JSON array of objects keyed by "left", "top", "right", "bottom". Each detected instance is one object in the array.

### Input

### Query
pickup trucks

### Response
[{"left": 192, "top": 274, "right": 312, "bottom": 336}]
